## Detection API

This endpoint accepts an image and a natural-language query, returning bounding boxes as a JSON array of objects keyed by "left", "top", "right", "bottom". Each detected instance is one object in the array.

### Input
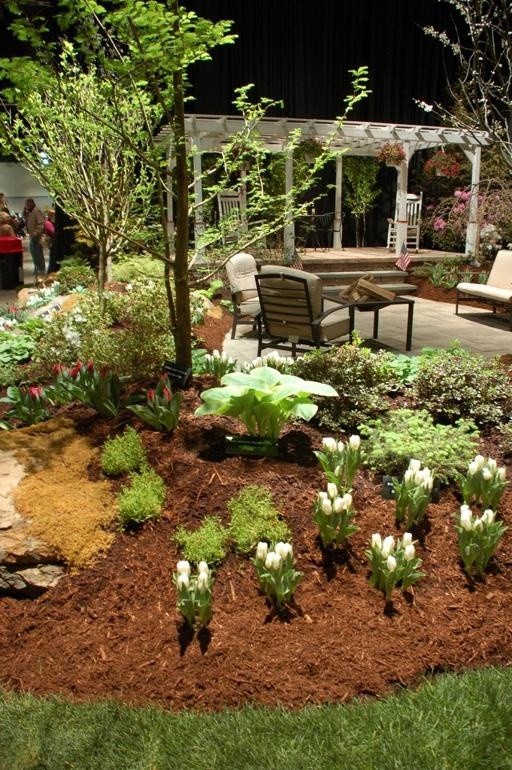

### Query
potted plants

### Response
[
  {"left": 229, "top": 147, "right": 259, "bottom": 170},
  {"left": 293, "top": 137, "right": 328, "bottom": 164}
]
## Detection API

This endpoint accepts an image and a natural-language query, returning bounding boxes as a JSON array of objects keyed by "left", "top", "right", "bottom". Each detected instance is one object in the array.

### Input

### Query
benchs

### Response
[{"left": 455, "top": 249, "right": 512, "bottom": 316}]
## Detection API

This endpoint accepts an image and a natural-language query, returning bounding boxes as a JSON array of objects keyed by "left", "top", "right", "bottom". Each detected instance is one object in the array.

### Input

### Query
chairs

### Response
[
  {"left": 290, "top": 211, "right": 346, "bottom": 252},
  {"left": 217, "top": 253, "right": 350, "bottom": 364},
  {"left": 217, "top": 191, "right": 267, "bottom": 251},
  {"left": 387, "top": 192, "right": 422, "bottom": 253}
]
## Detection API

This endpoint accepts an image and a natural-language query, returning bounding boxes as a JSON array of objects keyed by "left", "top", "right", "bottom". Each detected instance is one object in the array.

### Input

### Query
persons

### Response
[
  {"left": 23, "top": 199, "right": 46, "bottom": 276},
  {"left": 0, "top": 192, "right": 21, "bottom": 237}
]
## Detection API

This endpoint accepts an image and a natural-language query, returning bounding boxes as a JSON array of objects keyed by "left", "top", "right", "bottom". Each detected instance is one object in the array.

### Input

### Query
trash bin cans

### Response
[{"left": 0, "top": 235, "right": 24, "bottom": 290}]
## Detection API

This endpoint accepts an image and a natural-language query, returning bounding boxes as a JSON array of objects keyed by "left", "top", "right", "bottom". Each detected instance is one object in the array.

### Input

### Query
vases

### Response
[
  {"left": 385, "top": 155, "right": 399, "bottom": 167},
  {"left": 435, "top": 166, "right": 449, "bottom": 177}
]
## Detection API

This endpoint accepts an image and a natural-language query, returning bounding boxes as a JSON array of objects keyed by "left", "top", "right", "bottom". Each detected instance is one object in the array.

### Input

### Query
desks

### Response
[{"left": 312, "top": 289, "right": 415, "bottom": 351}]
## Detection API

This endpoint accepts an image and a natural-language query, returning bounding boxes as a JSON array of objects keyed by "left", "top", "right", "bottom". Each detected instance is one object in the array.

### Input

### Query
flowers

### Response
[
  {"left": 424, "top": 151, "right": 460, "bottom": 179},
  {"left": 379, "top": 142, "right": 407, "bottom": 166}
]
